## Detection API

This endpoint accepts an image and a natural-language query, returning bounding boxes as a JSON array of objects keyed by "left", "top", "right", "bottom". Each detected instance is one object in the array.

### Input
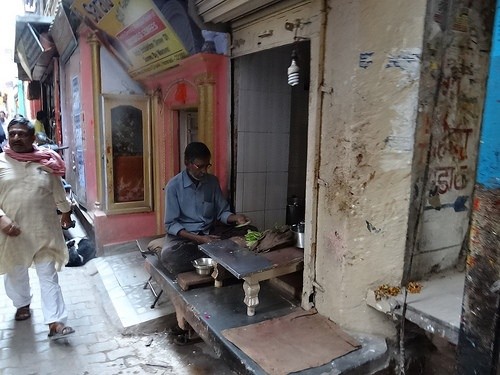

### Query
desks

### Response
[{"left": 198, "top": 235, "right": 303, "bottom": 317}]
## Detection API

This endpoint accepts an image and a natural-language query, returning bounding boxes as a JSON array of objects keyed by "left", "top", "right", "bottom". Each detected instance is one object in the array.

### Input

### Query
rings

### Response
[
  {"left": 9, "top": 228, "right": 12, "bottom": 231},
  {"left": 12, "top": 225, "right": 14, "bottom": 228}
]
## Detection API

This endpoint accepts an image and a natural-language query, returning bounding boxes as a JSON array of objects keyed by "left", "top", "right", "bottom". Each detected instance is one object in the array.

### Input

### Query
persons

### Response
[
  {"left": 161, "top": 142, "right": 258, "bottom": 274},
  {"left": 34, "top": 110, "right": 54, "bottom": 145},
  {"left": 0, "top": 111, "right": 9, "bottom": 140},
  {"left": 0, "top": 117, "right": 75, "bottom": 340}
]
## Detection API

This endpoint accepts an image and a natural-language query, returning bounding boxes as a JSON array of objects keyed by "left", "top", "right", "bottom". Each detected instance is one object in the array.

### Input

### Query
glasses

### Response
[{"left": 191, "top": 161, "right": 211, "bottom": 170}]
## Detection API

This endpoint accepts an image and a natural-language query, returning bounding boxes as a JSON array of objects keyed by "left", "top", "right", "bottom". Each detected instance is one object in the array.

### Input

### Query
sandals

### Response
[
  {"left": 15, "top": 305, "right": 30, "bottom": 321},
  {"left": 48, "top": 322, "right": 75, "bottom": 340}
]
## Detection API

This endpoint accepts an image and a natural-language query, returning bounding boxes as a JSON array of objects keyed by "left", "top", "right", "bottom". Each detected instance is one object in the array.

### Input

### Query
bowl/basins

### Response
[
  {"left": 192, "top": 257, "right": 214, "bottom": 276},
  {"left": 290, "top": 222, "right": 304, "bottom": 248}
]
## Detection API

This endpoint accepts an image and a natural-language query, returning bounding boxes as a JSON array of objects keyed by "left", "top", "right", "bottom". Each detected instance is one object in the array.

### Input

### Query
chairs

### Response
[{"left": 135, "top": 239, "right": 163, "bottom": 309}]
